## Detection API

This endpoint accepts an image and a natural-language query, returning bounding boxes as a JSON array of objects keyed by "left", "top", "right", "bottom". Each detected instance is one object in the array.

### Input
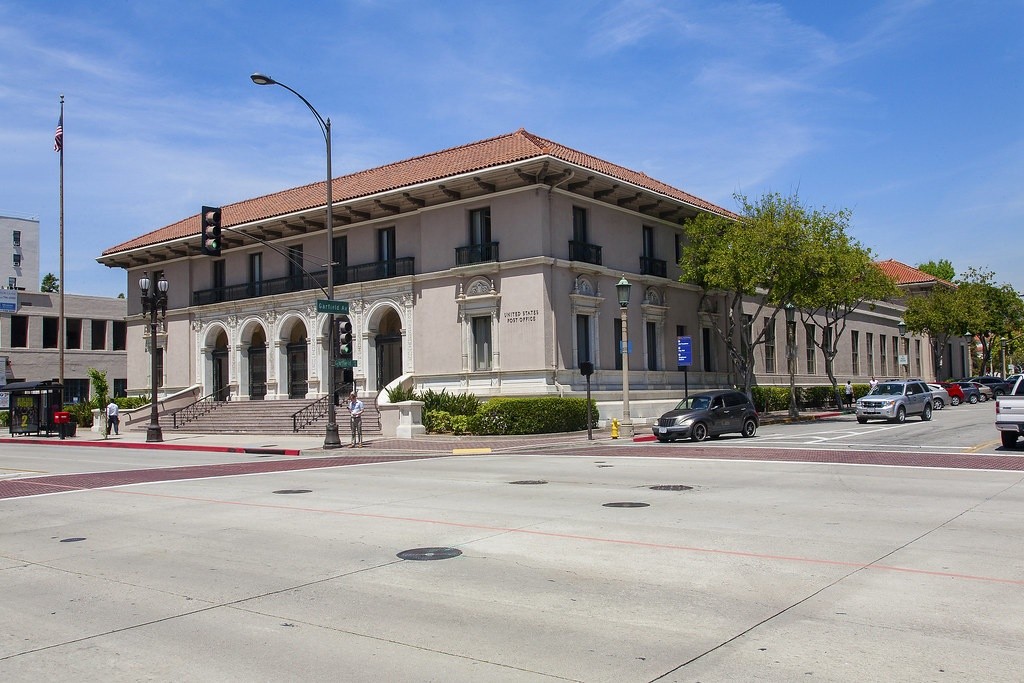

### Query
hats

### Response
[{"left": 110, "top": 399, "right": 114, "bottom": 402}]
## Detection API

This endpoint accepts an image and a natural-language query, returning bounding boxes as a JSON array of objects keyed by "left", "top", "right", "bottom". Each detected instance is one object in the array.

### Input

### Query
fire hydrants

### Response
[{"left": 610, "top": 418, "right": 620, "bottom": 439}]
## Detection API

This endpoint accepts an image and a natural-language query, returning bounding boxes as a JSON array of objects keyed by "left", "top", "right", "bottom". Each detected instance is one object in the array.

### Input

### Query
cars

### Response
[
  {"left": 959, "top": 374, "right": 1024, "bottom": 403},
  {"left": 928, "top": 383, "right": 964, "bottom": 406},
  {"left": 956, "top": 382, "right": 980, "bottom": 404},
  {"left": 652, "top": 389, "right": 760, "bottom": 443},
  {"left": 926, "top": 384, "right": 953, "bottom": 410}
]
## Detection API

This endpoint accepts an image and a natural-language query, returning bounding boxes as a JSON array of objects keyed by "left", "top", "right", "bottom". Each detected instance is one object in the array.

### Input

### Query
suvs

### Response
[{"left": 855, "top": 378, "right": 933, "bottom": 424}]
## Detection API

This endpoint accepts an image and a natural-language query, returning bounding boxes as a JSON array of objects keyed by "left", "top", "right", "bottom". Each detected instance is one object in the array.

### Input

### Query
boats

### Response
[{"left": 333, "top": 315, "right": 352, "bottom": 368}]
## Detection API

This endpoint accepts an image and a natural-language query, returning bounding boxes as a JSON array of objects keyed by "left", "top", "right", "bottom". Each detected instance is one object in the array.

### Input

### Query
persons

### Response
[
  {"left": 846, "top": 381, "right": 853, "bottom": 407},
  {"left": 106, "top": 399, "right": 118, "bottom": 435},
  {"left": 348, "top": 392, "right": 364, "bottom": 447},
  {"left": 870, "top": 376, "right": 878, "bottom": 389}
]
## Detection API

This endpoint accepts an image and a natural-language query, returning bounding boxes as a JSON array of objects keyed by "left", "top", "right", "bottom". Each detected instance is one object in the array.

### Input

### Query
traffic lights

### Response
[
  {"left": 580, "top": 362, "right": 594, "bottom": 376},
  {"left": 202, "top": 206, "right": 221, "bottom": 257}
]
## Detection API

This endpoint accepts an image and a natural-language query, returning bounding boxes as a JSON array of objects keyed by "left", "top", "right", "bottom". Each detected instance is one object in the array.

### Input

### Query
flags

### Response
[{"left": 53, "top": 115, "right": 63, "bottom": 151}]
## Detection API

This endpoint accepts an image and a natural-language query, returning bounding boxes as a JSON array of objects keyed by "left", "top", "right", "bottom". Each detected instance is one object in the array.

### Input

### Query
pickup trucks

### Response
[{"left": 995, "top": 375, "right": 1024, "bottom": 448}]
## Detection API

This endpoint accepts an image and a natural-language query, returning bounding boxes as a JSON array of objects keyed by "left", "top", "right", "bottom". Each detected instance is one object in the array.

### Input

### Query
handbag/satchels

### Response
[
  {"left": 852, "top": 398, "right": 855, "bottom": 402},
  {"left": 117, "top": 418, "right": 119, "bottom": 425}
]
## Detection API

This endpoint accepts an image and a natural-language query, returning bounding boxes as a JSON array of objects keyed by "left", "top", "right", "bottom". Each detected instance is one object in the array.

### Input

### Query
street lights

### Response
[
  {"left": 138, "top": 270, "right": 169, "bottom": 442},
  {"left": 614, "top": 274, "right": 634, "bottom": 438},
  {"left": 964, "top": 331, "right": 973, "bottom": 378},
  {"left": 1000, "top": 337, "right": 1007, "bottom": 380},
  {"left": 897, "top": 319, "right": 907, "bottom": 379},
  {"left": 783, "top": 303, "right": 799, "bottom": 417},
  {"left": 250, "top": 72, "right": 342, "bottom": 451}
]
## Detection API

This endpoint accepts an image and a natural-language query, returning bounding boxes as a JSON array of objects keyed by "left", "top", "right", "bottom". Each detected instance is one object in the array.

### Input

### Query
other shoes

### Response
[
  {"left": 115, "top": 434, "right": 118, "bottom": 435},
  {"left": 359, "top": 443, "right": 362, "bottom": 447},
  {"left": 105, "top": 433, "right": 110, "bottom": 435},
  {"left": 347, "top": 445, "right": 355, "bottom": 448}
]
